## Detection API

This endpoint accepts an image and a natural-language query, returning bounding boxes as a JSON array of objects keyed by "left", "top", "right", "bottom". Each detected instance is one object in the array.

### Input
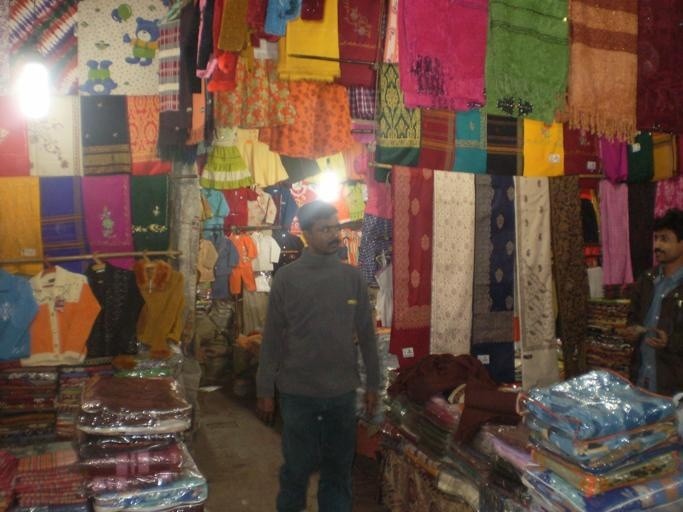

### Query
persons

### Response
[
  {"left": 258, "top": 202, "right": 381, "bottom": 512},
  {"left": 624, "top": 207, "right": 683, "bottom": 402}
]
